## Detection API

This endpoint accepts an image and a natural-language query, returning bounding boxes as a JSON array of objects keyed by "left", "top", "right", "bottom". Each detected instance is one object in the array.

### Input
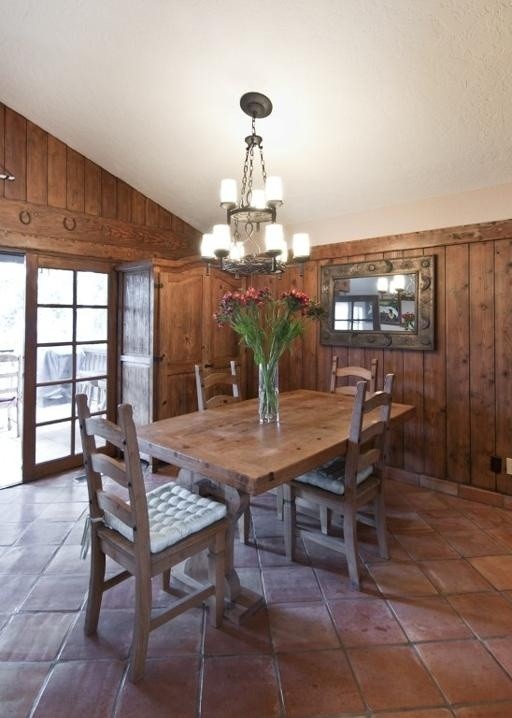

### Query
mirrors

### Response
[{"left": 319, "top": 254, "right": 435, "bottom": 349}]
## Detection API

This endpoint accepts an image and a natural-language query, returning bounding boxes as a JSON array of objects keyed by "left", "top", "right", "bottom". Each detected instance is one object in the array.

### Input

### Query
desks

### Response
[{"left": 134, "top": 388, "right": 417, "bottom": 625}]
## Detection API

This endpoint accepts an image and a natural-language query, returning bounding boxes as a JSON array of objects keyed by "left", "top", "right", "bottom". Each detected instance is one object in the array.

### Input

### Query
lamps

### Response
[
  {"left": 376, "top": 275, "right": 415, "bottom": 303},
  {"left": 194, "top": 92, "right": 311, "bottom": 278}
]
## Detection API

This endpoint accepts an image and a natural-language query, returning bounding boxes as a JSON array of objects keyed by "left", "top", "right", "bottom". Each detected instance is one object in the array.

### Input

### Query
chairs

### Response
[
  {"left": 71, "top": 348, "right": 110, "bottom": 415},
  {"left": 0, "top": 353, "right": 28, "bottom": 438}
]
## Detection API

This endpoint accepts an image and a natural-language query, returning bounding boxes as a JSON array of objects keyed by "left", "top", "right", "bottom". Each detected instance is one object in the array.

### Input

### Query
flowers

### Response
[
  {"left": 215, "top": 284, "right": 329, "bottom": 417},
  {"left": 399, "top": 311, "right": 418, "bottom": 331}
]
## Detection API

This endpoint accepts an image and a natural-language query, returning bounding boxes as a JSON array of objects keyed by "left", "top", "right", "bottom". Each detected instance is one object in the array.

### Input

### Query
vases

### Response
[{"left": 257, "top": 357, "right": 284, "bottom": 426}]
